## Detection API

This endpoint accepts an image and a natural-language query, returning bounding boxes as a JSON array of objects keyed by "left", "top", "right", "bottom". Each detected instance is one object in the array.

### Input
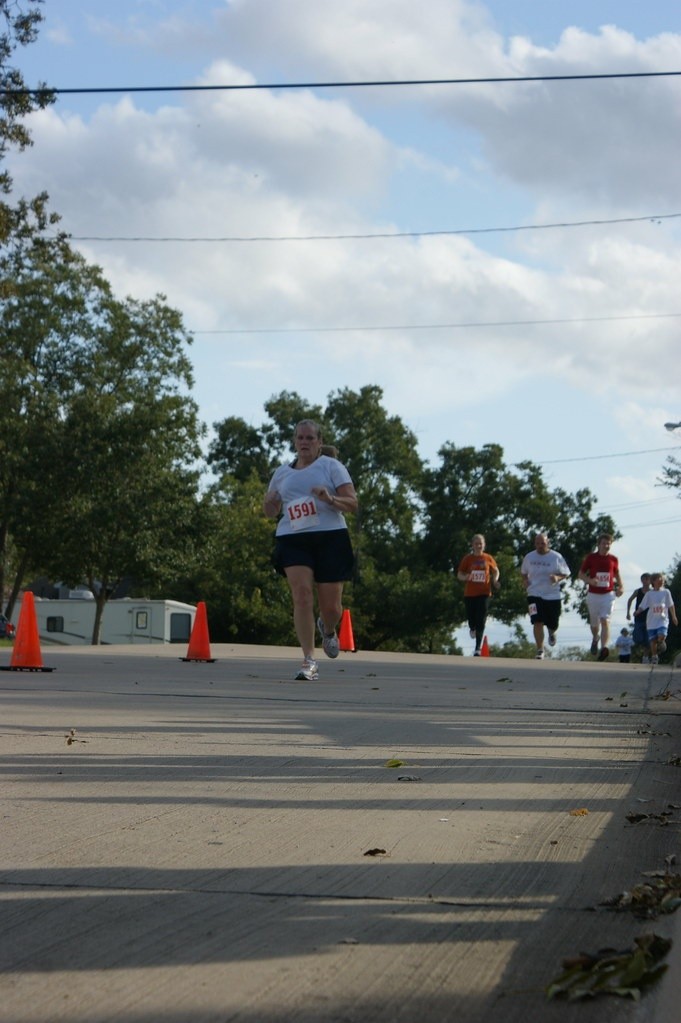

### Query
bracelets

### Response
[{"left": 328, "top": 496, "right": 334, "bottom": 506}]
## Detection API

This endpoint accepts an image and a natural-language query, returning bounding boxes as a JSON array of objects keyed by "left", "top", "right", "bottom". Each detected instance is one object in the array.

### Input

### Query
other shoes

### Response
[
  {"left": 474, "top": 649, "right": 482, "bottom": 656},
  {"left": 469, "top": 629, "right": 477, "bottom": 639}
]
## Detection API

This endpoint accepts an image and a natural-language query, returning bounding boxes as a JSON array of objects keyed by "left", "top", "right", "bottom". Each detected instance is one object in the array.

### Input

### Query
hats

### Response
[{"left": 620, "top": 627, "right": 629, "bottom": 634}]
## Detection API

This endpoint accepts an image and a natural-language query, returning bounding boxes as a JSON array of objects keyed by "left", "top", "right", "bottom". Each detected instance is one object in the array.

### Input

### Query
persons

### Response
[
  {"left": 264, "top": 419, "right": 358, "bottom": 681},
  {"left": 634, "top": 573, "right": 678, "bottom": 664},
  {"left": 627, "top": 574, "right": 652, "bottom": 664},
  {"left": 522, "top": 533, "right": 571, "bottom": 659},
  {"left": 615, "top": 629, "right": 635, "bottom": 663},
  {"left": 458, "top": 535, "right": 500, "bottom": 656},
  {"left": 578, "top": 534, "right": 623, "bottom": 661}
]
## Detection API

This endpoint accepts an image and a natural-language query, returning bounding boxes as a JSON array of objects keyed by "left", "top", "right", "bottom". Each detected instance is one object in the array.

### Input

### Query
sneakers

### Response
[
  {"left": 535, "top": 649, "right": 545, "bottom": 659},
  {"left": 548, "top": 630, "right": 557, "bottom": 646},
  {"left": 597, "top": 647, "right": 610, "bottom": 661},
  {"left": 651, "top": 655, "right": 659, "bottom": 668},
  {"left": 642, "top": 656, "right": 650, "bottom": 664},
  {"left": 591, "top": 635, "right": 600, "bottom": 655},
  {"left": 317, "top": 616, "right": 340, "bottom": 660},
  {"left": 295, "top": 656, "right": 319, "bottom": 680}
]
